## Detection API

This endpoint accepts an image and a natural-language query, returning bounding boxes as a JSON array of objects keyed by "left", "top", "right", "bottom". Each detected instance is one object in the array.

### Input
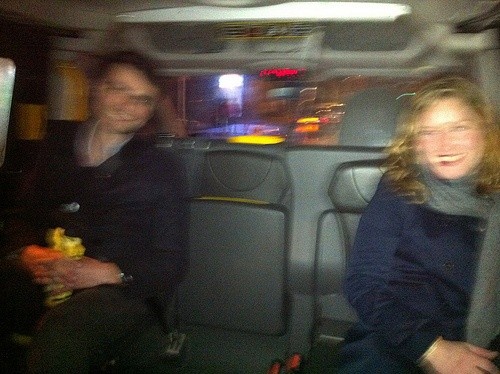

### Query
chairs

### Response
[
  {"left": 307, "top": 159, "right": 388, "bottom": 358},
  {"left": 170, "top": 148, "right": 293, "bottom": 373}
]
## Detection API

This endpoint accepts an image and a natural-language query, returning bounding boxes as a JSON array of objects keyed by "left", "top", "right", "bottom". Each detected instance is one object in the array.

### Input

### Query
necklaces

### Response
[{"left": 82, "top": 112, "right": 131, "bottom": 165}]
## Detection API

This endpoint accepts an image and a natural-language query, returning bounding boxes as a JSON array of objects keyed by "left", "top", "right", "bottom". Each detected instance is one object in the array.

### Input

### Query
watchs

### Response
[{"left": 112, "top": 259, "right": 135, "bottom": 289}]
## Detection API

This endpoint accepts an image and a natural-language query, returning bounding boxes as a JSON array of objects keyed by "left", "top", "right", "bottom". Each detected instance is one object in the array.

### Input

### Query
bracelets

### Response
[{"left": 415, "top": 335, "right": 443, "bottom": 368}]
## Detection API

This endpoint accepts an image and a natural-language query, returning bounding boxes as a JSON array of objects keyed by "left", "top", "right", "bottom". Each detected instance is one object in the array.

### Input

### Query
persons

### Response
[
  {"left": 1, "top": 49, "right": 187, "bottom": 373},
  {"left": 315, "top": 74, "right": 500, "bottom": 373}
]
